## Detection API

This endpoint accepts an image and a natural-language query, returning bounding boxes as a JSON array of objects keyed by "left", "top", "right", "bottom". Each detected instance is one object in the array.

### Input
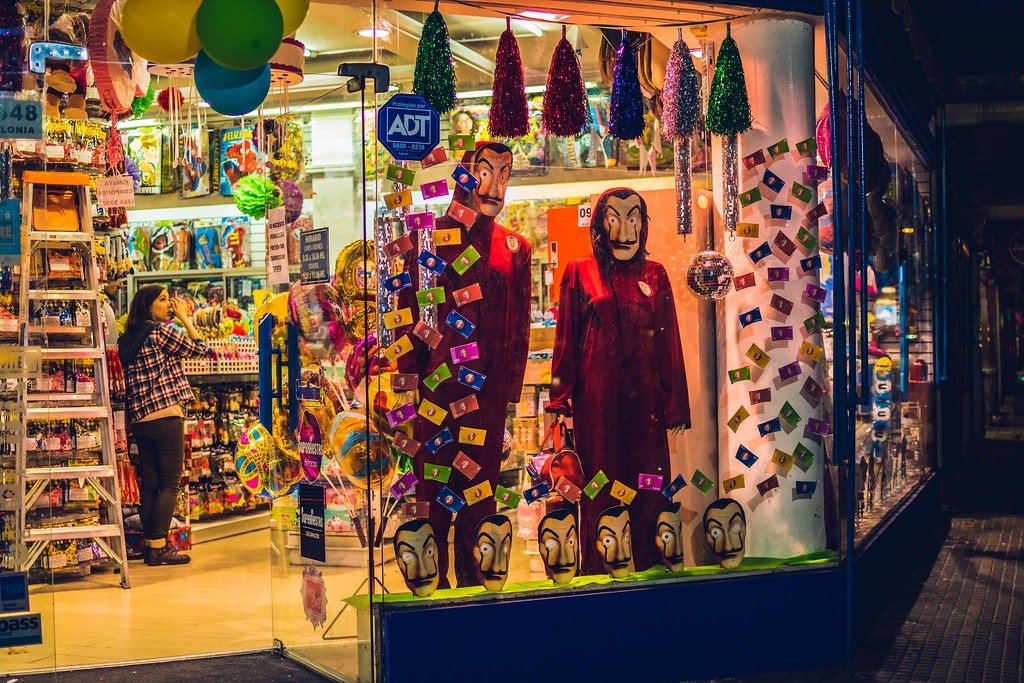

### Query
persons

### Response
[
  {"left": 118, "top": 286, "right": 207, "bottom": 567},
  {"left": 395, "top": 142, "right": 533, "bottom": 591},
  {"left": 544, "top": 188, "right": 692, "bottom": 579}
]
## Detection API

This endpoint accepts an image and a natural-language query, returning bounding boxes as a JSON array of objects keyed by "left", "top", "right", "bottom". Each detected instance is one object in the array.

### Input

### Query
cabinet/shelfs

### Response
[
  {"left": 185, "top": 374, "right": 272, "bottom": 540},
  {"left": 589, "top": 188, "right": 717, "bottom": 567}
]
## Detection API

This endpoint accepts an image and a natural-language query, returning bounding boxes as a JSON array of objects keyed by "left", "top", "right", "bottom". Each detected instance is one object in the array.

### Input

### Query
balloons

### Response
[{"left": 120, "top": 0, "right": 311, "bottom": 116}]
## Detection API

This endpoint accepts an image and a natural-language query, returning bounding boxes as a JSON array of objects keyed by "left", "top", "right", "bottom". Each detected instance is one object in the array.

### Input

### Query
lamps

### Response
[{"left": 877, "top": 197, "right": 914, "bottom": 233}]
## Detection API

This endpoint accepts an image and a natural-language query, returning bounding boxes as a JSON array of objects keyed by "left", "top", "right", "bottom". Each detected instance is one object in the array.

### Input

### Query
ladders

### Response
[{"left": 13, "top": 170, "right": 131, "bottom": 589}]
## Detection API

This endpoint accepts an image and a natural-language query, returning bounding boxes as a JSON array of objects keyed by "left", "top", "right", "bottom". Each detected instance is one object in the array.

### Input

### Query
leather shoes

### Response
[{"left": 144, "top": 544, "right": 191, "bottom": 566}]
